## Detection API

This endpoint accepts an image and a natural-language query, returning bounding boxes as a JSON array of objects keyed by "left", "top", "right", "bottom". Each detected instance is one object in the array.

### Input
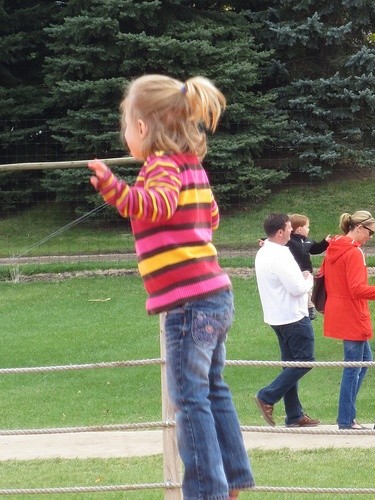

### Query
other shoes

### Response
[{"left": 339, "top": 423, "right": 364, "bottom": 430}]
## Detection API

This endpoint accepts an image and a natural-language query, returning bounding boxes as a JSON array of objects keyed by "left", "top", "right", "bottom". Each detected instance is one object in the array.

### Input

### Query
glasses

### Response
[{"left": 356, "top": 223, "right": 375, "bottom": 236}]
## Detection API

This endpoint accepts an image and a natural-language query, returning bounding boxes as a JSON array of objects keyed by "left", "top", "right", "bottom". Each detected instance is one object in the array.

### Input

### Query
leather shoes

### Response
[
  {"left": 255, "top": 395, "right": 276, "bottom": 427},
  {"left": 286, "top": 413, "right": 321, "bottom": 427}
]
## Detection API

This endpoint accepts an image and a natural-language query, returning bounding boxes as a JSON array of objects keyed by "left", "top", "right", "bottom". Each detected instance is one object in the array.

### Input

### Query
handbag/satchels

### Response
[{"left": 311, "top": 274, "right": 327, "bottom": 315}]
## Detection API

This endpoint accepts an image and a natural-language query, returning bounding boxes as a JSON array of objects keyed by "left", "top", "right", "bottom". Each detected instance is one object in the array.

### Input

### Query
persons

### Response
[
  {"left": 323, "top": 210, "right": 375, "bottom": 430},
  {"left": 89, "top": 73, "right": 258, "bottom": 500},
  {"left": 252, "top": 214, "right": 321, "bottom": 429},
  {"left": 259, "top": 214, "right": 332, "bottom": 322}
]
